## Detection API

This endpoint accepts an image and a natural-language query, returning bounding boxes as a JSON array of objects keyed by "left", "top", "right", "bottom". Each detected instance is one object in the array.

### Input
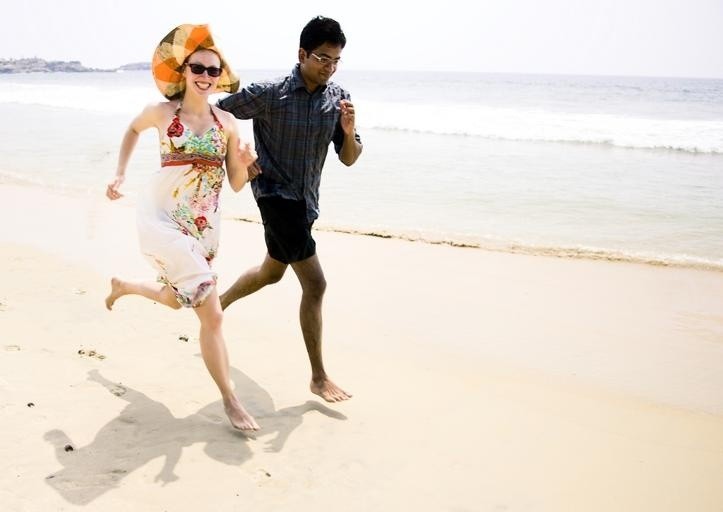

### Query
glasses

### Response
[
  {"left": 309, "top": 51, "right": 342, "bottom": 68},
  {"left": 182, "top": 61, "right": 223, "bottom": 78}
]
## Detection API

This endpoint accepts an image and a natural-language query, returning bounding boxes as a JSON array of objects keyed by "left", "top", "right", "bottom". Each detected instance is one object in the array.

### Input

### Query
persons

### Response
[
  {"left": 207, "top": 15, "right": 364, "bottom": 404},
  {"left": 101, "top": 22, "right": 260, "bottom": 432}
]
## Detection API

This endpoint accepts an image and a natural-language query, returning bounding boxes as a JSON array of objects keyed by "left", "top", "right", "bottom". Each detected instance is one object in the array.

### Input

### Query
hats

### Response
[{"left": 148, "top": 23, "right": 241, "bottom": 101}]
